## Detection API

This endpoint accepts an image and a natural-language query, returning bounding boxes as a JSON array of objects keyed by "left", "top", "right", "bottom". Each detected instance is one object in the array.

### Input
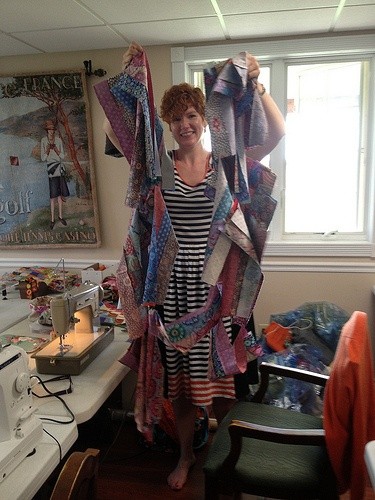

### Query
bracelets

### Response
[{"left": 259, "top": 83, "right": 266, "bottom": 97}]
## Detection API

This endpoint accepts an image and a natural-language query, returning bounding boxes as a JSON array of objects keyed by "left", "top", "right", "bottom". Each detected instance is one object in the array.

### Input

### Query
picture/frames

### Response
[{"left": 0, "top": 71, "right": 103, "bottom": 248}]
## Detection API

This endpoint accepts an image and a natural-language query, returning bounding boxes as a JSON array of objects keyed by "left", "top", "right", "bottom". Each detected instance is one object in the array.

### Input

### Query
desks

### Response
[
  {"left": 0, "top": 416, "right": 78, "bottom": 500},
  {"left": 0, "top": 298, "right": 132, "bottom": 425}
]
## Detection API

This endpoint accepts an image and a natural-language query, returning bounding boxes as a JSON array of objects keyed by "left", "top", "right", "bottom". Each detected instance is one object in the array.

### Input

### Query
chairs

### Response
[{"left": 200, "top": 311, "right": 375, "bottom": 500}]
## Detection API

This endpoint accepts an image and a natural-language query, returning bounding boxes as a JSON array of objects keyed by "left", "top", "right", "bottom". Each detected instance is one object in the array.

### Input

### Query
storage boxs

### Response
[{"left": 81, "top": 263, "right": 113, "bottom": 283}]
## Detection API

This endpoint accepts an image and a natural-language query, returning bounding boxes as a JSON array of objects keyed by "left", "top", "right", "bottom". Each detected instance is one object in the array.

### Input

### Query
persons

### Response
[{"left": 116, "top": 40, "right": 286, "bottom": 491}]
